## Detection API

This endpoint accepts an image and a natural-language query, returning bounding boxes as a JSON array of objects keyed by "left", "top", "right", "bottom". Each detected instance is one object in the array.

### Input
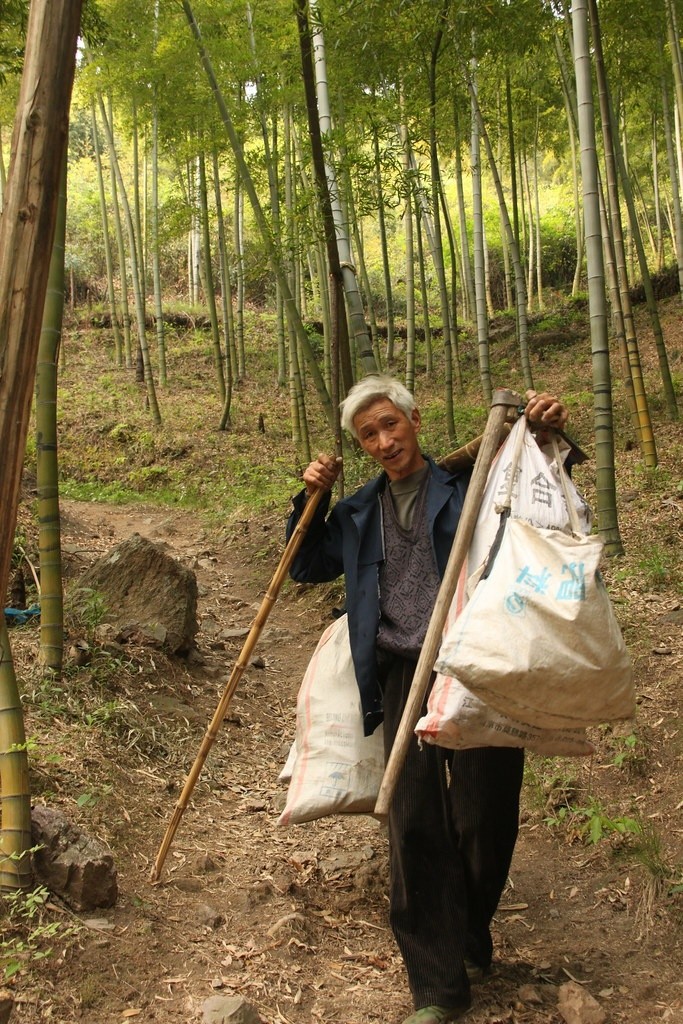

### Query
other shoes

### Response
[
  {"left": 401, "top": 1003, "right": 457, "bottom": 1024},
  {"left": 463, "top": 957, "right": 482, "bottom": 977}
]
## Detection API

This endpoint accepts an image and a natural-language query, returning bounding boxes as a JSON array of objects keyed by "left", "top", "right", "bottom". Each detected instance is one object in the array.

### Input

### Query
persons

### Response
[{"left": 286, "top": 374, "right": 573, "bottom": 1024}]
